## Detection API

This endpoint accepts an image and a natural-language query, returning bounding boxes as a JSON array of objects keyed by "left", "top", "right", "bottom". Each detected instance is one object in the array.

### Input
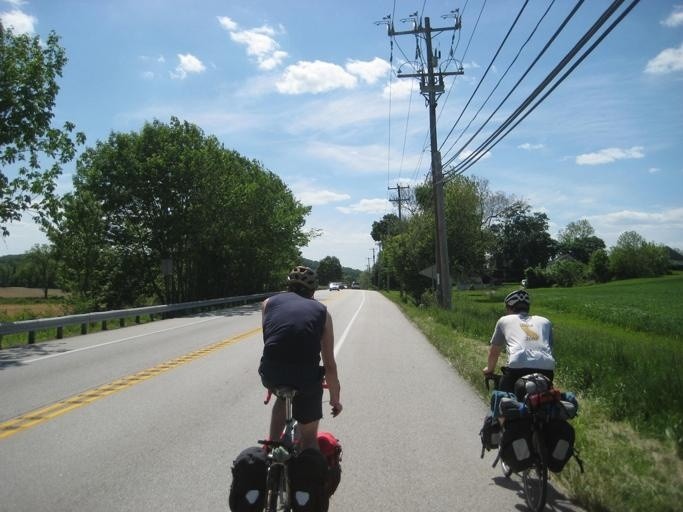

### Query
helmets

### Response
[
  {"left": 286, "top": 264, "right": 320, "bottom": 292},
  {"left": 504, "top": 289, "right": 531, "bottom": 307}
]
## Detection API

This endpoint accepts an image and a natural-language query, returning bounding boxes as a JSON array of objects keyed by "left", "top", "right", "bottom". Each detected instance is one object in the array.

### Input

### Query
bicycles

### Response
[
  {"left": 482, "top": 369, "right": 549, "bottom": 511},
  {"left": 256, "top": 384, "right": 342, "bottom": 511}
]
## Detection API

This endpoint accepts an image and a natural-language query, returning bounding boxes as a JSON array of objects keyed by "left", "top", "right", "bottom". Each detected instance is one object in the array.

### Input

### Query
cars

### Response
[{"left": 328, "top": 281, "right": 360, "bottom": 291}]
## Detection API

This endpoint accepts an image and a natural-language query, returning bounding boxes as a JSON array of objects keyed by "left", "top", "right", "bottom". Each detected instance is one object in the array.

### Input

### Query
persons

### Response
[
  {"left": 255, "top": 264, "right": 342, "bottom": 457},
  {"left": 482, "top": 289, "right": 556, "bottom": 398}
]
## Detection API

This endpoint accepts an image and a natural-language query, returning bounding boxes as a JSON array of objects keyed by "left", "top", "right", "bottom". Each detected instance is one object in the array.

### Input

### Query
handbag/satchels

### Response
[
  {"left": 479, "top": 371, "right": 580, "bottom": 475},
  {"left": 287, "top": 432, "right": 344, "bottom": 512},
  {"left": 228, "top": 444, "right": 270, "bottom": 512}
]
